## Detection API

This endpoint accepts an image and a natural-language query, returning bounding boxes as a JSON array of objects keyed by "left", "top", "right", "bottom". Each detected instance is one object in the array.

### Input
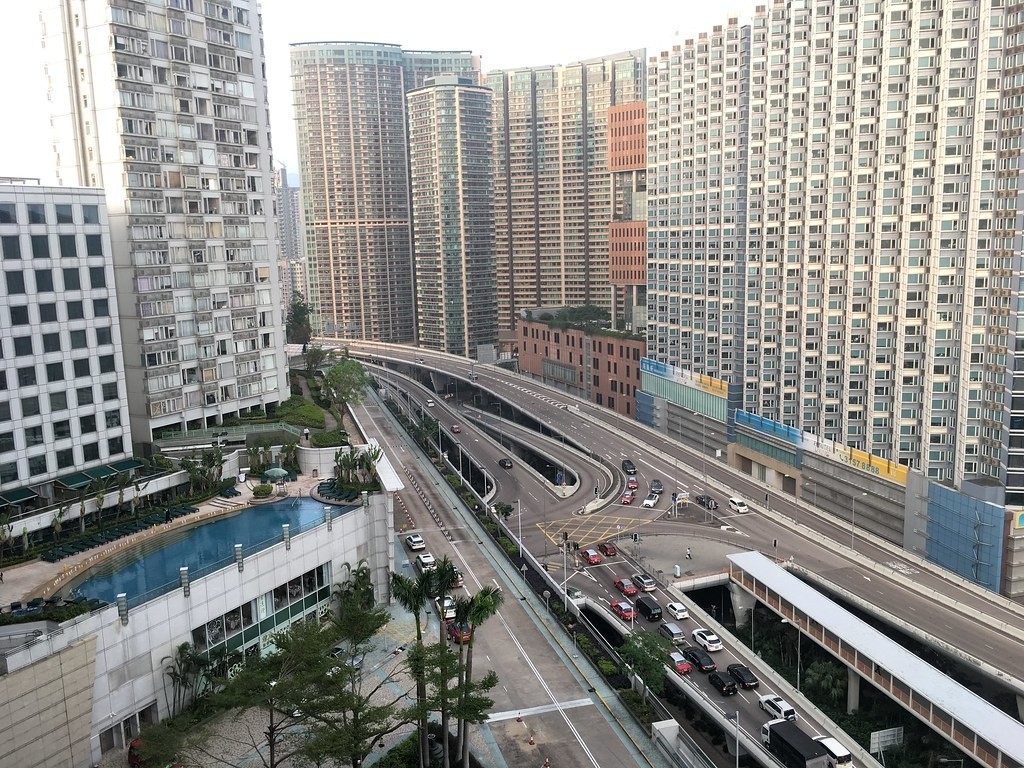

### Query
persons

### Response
[
  {"left": 304, "top": 427, "right": 310, "bottom": 440},
  {"left": 164, "top": 506, "right": 172, "bottom": 525},
  {"left": 686, "top": 548, "right": 692, "bottom": 559},
  {"left": 573, "top": 540, "right": 580, "bottom": 551}
]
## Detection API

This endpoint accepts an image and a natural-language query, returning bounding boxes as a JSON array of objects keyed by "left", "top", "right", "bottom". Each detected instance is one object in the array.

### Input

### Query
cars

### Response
[
  {"left": 451, "top": 424, "right": 460, "bottom": 434},
  {"left": 695, "top": 494, "right": 718, "bottom": 510},
  {"left": 468, "top": 372, "right": 478, "bottom": 380},
  {"left": 264, "top": 646, "right": 365, "bottom": 717},
  {"left": 499, "top": 458, "right": 512, "bottom": 470},
  {"left": 128, "top": 738, "right": 185, "bottom": 768},
  {"left": 611, "top": 572, "right": 761, "bottom": 698},
  {"left": 415, "top": 357, "right": 425, "bottom": 365},
  {"left": 643, "top": 479, "right": 666, "bottom": 508},
  {"left": 425, "top": 399, "right": 435, "bottom": 408},
  {"left": 582, "top": 549, "right": 601, "bottom": 565},
  {"left": 621, "top": 460, "right": 638, "bottom": 504},
  {"left": 598, "top": 543, "right": 618, "bottom": 557},
  {"left": 404, "top": 533, "right": 474, "bottom": 644}
]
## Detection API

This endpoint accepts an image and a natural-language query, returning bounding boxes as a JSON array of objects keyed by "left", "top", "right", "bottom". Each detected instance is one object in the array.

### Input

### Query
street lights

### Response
[
  {"left": 455, "top": 432, "right": 487, "bottom": 519},
  {"left": 490, "top": 403, "right": 503, "bottom": 444},
  {"left": 781, "top": 618, "right": 802, "bottom": 692},
  {"left": 436, "top": 419, "right": 442, "bottom": 463},
  {"left": 513, "top": 499, "right": 523, "bottom": 558},
  {"left": 940, "top": 758, "right": 964, "bottom": 768},
  {"left": 419, "top": 404, "right": 424, "bottom": 430},
  {"left": 694, "top": 412, "right": 708, "bottom": 522},
  {"left": 784, "top": 473, "right": 867, "bottom": 552},
  {"left": 376, "top": 362, "right": 411, "bottom": 424}
]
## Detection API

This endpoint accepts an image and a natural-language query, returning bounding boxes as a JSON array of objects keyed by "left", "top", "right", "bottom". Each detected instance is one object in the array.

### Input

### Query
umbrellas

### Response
[{"left": 264, "top": 467, "right": 288, "bottom": 494}]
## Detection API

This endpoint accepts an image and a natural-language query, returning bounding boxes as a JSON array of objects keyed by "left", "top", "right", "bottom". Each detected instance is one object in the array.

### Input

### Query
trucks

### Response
[{"left": 761, "top": 718, "right": 828, "bottom": 768}]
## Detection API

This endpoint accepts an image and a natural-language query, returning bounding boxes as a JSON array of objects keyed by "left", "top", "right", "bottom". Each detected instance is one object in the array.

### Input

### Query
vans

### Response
[
  {"left": 758, "top": 694, "right": 798, "bottom": 724},
  {"left": 728, "top": 498, "right": 749, "bottom": 514},
  {"left": 810, "top": 735, "right": 853, "bottom": 768}
]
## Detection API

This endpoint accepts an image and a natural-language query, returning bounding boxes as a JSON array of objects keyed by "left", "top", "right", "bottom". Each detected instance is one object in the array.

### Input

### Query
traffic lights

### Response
[
  {"left": 567, "top": 543, "right": 571, "bottom": 550},
  {"left": 630, "top": 534, "right": 634, "bottom": 540},
  {"left": 572, "top": 541, "right": 580, "bottom": 551},
  {"left": 633, "top": 533, "right": 639, "bottom": 544}
]
